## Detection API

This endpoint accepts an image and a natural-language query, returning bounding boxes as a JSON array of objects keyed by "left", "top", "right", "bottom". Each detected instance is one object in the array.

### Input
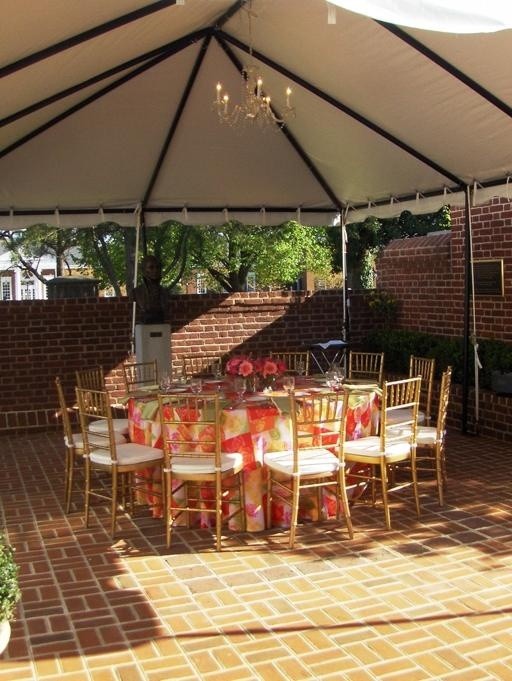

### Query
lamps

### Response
[{"left": 210, "top": 1, "right": 298, "bottom": 137}]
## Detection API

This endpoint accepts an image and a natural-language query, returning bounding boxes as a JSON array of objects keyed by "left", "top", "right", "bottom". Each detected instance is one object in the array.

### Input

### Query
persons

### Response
[{"left": 130, "top": 255, "right": 177, "bottom": 326}]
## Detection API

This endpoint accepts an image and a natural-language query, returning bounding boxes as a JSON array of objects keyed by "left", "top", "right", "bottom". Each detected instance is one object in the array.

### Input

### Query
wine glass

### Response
[{"left": 157, "top": 356, "right": 346, "bottom": 402}]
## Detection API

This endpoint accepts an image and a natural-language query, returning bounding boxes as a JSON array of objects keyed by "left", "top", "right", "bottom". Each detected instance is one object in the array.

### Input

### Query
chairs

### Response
[{"left": 55, "top": 347, "right": 452, "bottom": 551}]
